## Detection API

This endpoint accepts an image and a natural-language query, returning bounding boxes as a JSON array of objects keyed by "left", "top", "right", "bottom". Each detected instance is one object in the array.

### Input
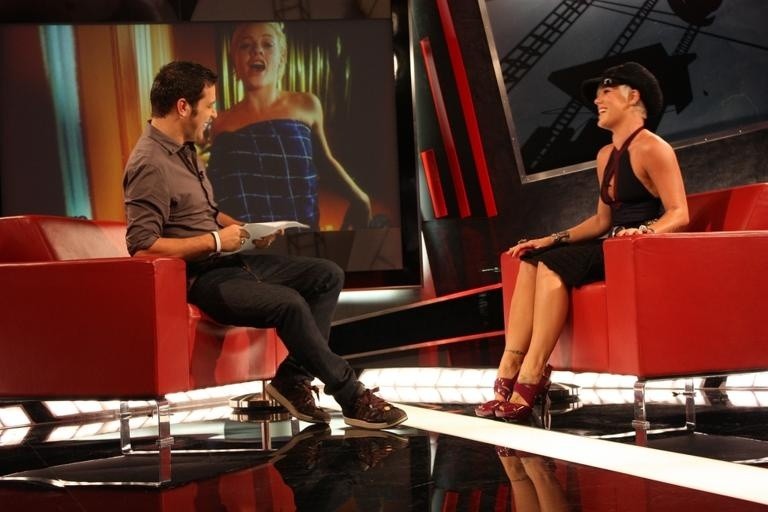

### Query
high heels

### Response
[
  {"left": 493, "top": 364, "right": 555, "bottom": 421},
  {"left": 474, "top": 365, "right": 521, "bottom": 420}
]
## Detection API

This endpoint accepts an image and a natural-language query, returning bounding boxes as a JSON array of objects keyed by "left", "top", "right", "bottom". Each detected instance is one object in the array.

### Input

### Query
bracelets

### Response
[
  {"left": 209, "top": 231, "right": 220, "bottom": 255},
  {"left": 639, "top": 223, "right": 655, "bottom": 236}
]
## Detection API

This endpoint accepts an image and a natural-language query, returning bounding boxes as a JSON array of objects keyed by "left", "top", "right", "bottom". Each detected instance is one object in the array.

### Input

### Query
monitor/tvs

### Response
[
  {"left": 0, "top": 0, "right": 425, "bottom": 292},
  {"left": 477, "top": 0, "right": 768, "bottom": 185}
]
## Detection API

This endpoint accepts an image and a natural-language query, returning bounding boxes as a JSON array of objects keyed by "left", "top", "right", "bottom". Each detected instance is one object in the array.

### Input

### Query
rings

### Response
[{"left": 240, "top": 237, "right": 244, "bottom": 245}]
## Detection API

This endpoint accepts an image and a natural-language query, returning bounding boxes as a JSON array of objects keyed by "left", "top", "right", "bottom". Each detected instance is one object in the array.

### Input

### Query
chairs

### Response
[
  {"left": 499, "top": 182, "right": 767, "bottom": 426},
  {"left": 0, "top": 214, "right": 300, "bottom": 448}
]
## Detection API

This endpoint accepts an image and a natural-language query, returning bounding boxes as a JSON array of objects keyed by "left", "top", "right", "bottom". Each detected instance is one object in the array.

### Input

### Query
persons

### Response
[
  {"left": 207, "top": 22, "right": 373, "bottom": 237},
  {"left": 270, "top": 424, "right": 410, "bottom": 512},
  {"left": 474, "top": 63, "right": 691, "bottom": 417},
  {"left": 494, "top": 445, "right": 571, "bottom": 512},
  {"left": 122, "top": 60, "right": 408, "bottom": 432}
]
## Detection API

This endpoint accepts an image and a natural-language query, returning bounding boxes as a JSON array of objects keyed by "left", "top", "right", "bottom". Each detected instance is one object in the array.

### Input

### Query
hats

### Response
[{"left": 580, "top": 60, "right": 665, "bottom": 120}]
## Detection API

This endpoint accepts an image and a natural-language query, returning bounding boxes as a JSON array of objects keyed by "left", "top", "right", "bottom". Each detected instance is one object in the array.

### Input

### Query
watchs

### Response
[{"left": 551, "top": 230, "right": 569, "bottom": 246}]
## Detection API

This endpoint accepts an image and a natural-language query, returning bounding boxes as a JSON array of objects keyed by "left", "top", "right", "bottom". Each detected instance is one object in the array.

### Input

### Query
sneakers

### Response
[
  {"left": 343, "top": 426, "right": 411, "bottom": 468},
  {"left": 262, "top": 372, "right": 331, "bottom": 425},
  {"left": 268, "top": 424, "right": 331, "bottom": 486},
  {"left": 340, "top": 383, "right": 408, "bottom": 430}
]
